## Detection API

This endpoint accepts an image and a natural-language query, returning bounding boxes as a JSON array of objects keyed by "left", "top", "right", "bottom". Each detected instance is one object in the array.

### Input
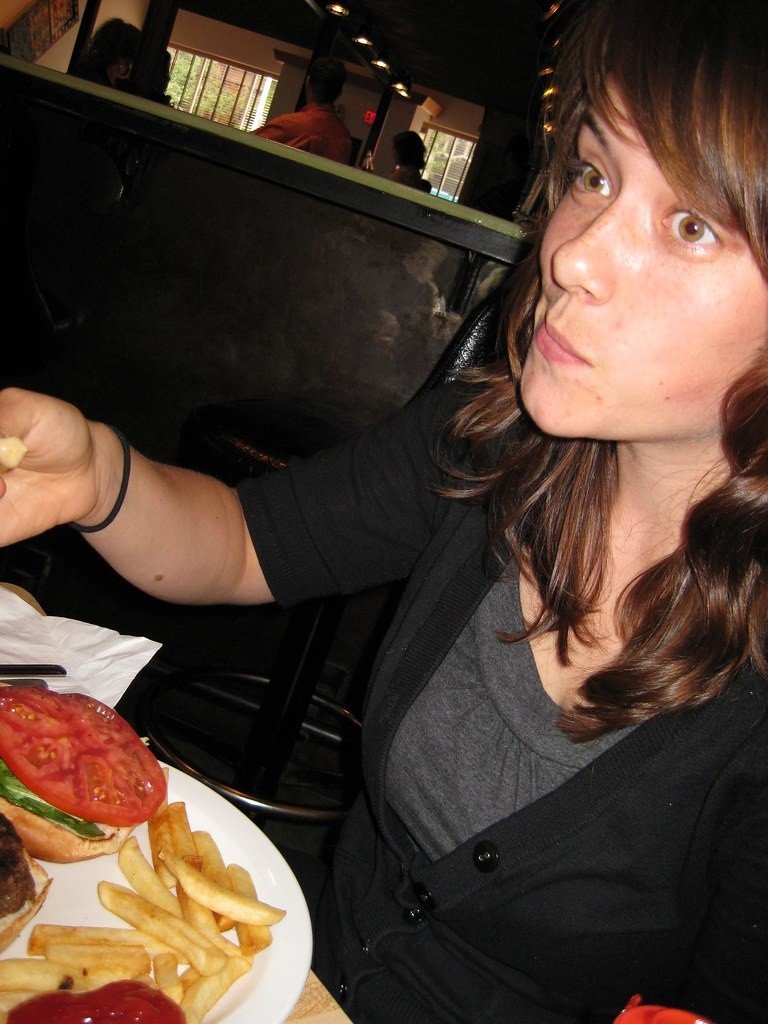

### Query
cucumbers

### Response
[{"left": 0, "top": 758, "right": 108, "bottom": 839}]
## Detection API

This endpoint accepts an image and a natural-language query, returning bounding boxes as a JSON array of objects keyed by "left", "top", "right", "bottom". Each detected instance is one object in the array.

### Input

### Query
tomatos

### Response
[{"left": 0, "top": 686, "right": 167, "bottom": 827}]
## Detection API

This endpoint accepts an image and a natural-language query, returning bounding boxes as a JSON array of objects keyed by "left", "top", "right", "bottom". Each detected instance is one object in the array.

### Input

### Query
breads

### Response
[{"left": 0, "top": 812, "right": 52, "bottom": 953}]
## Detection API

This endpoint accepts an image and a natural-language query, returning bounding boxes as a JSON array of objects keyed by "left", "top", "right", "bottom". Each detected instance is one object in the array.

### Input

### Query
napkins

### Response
[{"left": 0, "top": 586, "right": 163, "bottom": 709}]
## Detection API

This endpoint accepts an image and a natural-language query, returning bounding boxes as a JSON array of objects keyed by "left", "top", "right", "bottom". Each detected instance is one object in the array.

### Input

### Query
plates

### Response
[{"left": 0, "top": 759, "right": 314, "bottom": 1023}]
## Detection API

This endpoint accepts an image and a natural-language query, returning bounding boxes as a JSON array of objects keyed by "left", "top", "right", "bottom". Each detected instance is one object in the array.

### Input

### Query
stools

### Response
[{"left": 132, "top": 394, "right": 387, "bottom": 829}]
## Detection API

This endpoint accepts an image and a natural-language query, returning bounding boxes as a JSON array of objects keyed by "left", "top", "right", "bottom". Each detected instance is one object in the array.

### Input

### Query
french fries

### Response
[
  {"left": 0, "top": 768, "right": 287, "bottom": 1024},
  {"left": 0, "top": 435, "right": 33, "bottom": 470}
]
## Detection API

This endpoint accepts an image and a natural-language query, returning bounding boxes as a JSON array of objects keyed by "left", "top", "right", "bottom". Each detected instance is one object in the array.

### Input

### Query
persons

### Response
[
  {"left": 386, "top": 131, "right": 427, "bottom": 187},
  {"left": 249, "top": 58, "right": 353, "bottom": 164},
  {"left": 0, "top": 0, "right": 767, "bottom": 1024},
  {"left": 68, "top": 18, "right": 173, "bottom": 107}
]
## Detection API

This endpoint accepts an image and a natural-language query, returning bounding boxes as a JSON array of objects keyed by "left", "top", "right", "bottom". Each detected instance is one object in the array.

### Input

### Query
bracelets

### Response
[{"left": 67, "top": 426, "right": 132, "bottom": 533}]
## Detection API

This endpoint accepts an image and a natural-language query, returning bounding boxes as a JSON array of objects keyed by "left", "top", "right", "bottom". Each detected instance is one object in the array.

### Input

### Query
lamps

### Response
[
  {"left": 352, "top": 23, "right": 374, "bottom": 46},
  {"left": 390, "top": 70, "right": 412, "bottom": 91},
  {"left": 371, "top": 49, "right": 391, "bottom": 70},
  {"left": 325, "top": 0, "right": 350, "bottom": 16}
]
